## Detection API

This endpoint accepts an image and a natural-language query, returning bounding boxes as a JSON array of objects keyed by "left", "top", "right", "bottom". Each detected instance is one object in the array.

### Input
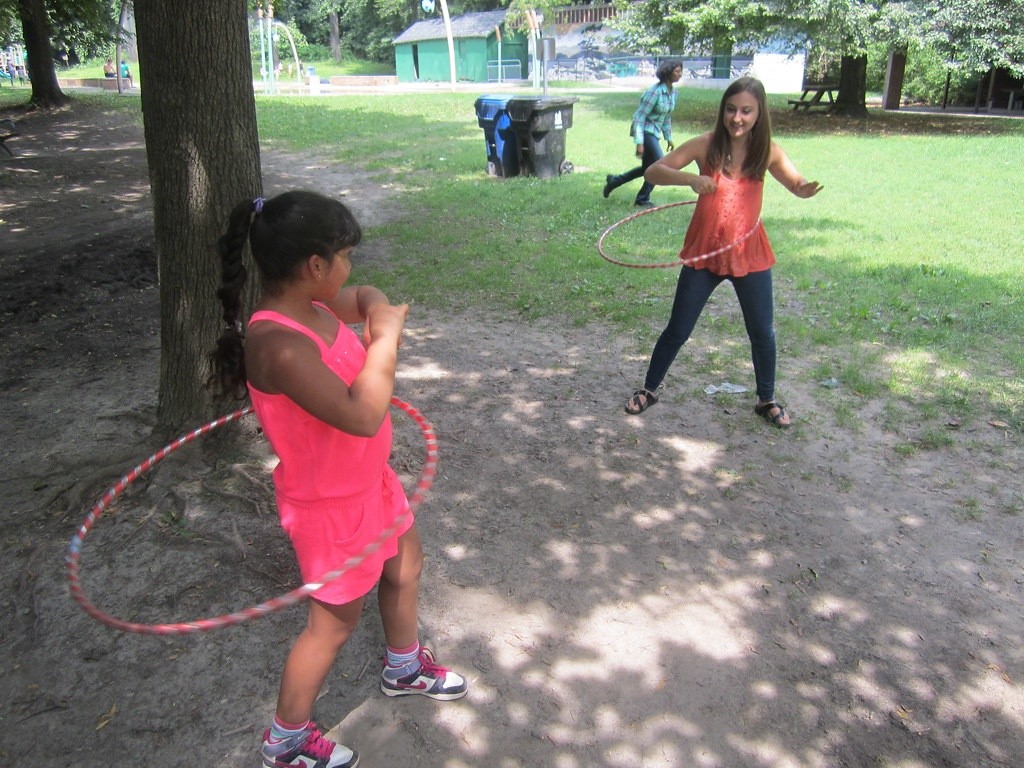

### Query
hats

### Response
[{"left": 656, "top": 62, "right": 683, "bottom": 81}]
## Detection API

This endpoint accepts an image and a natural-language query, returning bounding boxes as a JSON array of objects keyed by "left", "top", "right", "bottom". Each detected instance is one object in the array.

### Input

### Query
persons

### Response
[
  {"left": 104, "top": 58, "right": 117, "bottom": 78},
  {"left": 120, "top": 61, "right": 136, "bottom": 88},
  {"left": 207, "top": 189, "right": 471, "bottom": 768},
  {"left": 18, "top": 66, "right": 25, "bottom": 87},
  {"left": 624, "top": 75, "right": 826, "bottom": 429},
  {"left": 7, "top": 57, "right": 15, "bottom": 87},
  {"left": 603, "top": 58, "right": 684, "bottom": 209}
]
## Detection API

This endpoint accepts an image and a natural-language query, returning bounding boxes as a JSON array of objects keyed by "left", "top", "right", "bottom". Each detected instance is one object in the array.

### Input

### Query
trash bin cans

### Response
[
  {"left": 309, "top": 67, "right": 315, "bottom": 74},
  {"left": 474, "top": 94, "right": 536, "bottom": 178},
  {"left": 505, "top": 96, "right": 577, "bottom": 178}
]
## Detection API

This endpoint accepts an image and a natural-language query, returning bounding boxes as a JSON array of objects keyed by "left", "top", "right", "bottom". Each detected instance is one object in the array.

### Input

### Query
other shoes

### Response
[
  {"left": 604, "top": 175, "right": 616, "bottom": 197},
  {"left": 634, "top": 200, "right": 656, "bottom": 208}
]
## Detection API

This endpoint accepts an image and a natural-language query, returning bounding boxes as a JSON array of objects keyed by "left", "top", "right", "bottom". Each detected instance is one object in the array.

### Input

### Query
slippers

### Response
[
  {"left": 754, "top": 402, "right": 790, "bottom": 427},
  {"left": 625, "top": 390, "right": 658, "bottom": 414}
]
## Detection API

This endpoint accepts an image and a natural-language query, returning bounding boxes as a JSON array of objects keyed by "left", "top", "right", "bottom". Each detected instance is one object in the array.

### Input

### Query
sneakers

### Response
[
  {"left": 380, "top": 646, "right": 467, "bottom": 700},
  {"left": 261, "top": 722, "right": 360, "bottom": 768}
]
